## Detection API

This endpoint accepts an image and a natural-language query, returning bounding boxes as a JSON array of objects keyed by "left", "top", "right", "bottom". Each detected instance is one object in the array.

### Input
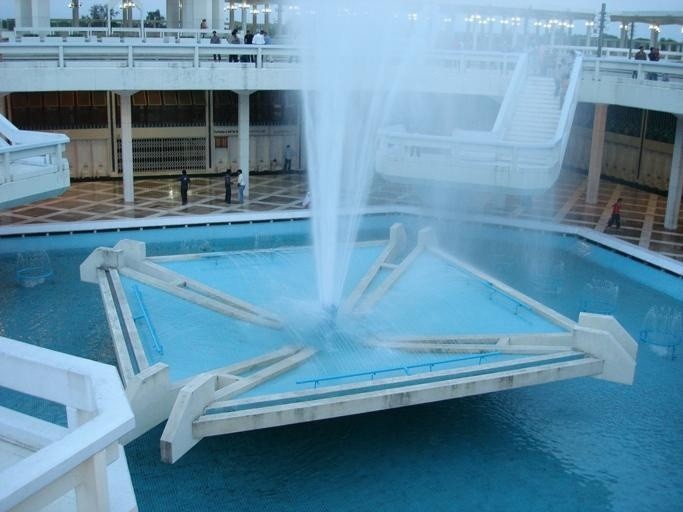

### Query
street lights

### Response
[
  {"left": 69, "top": 0, "right": 82, "bottom": 27},
  {"left": 281, "top": 5, "right": 573, "bottom": 45},
  {"left": 583, "top": 17, "right": 596, "bottom": 44},
  {"left": 617, "top": 19, "right": 629, "bottom": 48},
  {"left": 648, "top": 22, "right": 660, "bottom": 48},
  {"left": 261, "top": 0, "right": 270, "bottom": 33},
  {"left": 224, "top": 1, "right": 237, "bottom": 30},
  {"left": 119, "top": 0, "right": 135, "bottom": 28},
  {"left": 249, "top": 0, "right": 260, "bottom": 33},
  {"left": 238, "top": 0, "right": 250, "bottom": 33}
]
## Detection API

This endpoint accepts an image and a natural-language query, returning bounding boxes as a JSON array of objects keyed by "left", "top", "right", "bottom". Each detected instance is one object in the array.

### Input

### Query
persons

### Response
[
  {"left": 224, "top": 169, "right": 235, "bottom": 203},
  {"left": 200, "top": 18, "right": 271, "bottom": 62},
  {"left": 553, "top": 50, "right": 575, "bottom": 109},
  {"left": 632, "top": 47, "right": 660, "bottom": 80},
  {"left": 175, "top": 169, "right": 191, "bottom": 205},
  {"left": 606, "top": 197, "right": 623, "bottom": 230},
  {"left": 237, "top": 170, "right": 246, "bottom": 201},
  {"left": 282, "top": 144, "right": 296, "bottom": 172}
]
